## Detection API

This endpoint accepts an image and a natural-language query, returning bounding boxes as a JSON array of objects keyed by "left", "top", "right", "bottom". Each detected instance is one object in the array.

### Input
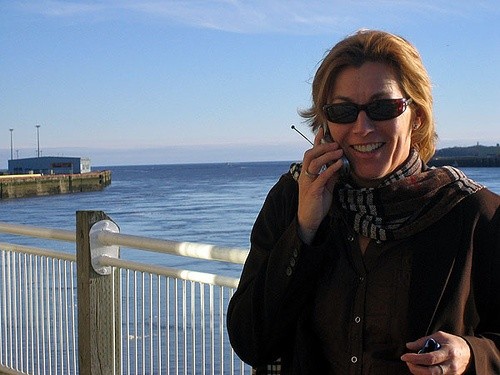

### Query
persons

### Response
[{"left": 224, "top": 28, "right": 499, "bottom": 375}]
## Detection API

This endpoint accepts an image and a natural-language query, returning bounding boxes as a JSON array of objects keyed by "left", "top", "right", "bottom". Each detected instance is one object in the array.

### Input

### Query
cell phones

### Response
[{"left": 320, "top": 121, "right": 350, "bottom": 177}]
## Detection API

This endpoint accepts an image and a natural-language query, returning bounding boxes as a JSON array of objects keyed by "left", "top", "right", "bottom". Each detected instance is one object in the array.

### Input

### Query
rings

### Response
[
  {"left": 438, "top": 365, "right": 447, "bottom": 375},
  {"left": 304, "top": 169, "right": 319, "bottom": 181}
]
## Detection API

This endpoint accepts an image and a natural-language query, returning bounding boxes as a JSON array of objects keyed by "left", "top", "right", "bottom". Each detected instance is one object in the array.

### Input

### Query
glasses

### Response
[{"left": 321, "top": 98, "right": 413, "bottom": 123}]
